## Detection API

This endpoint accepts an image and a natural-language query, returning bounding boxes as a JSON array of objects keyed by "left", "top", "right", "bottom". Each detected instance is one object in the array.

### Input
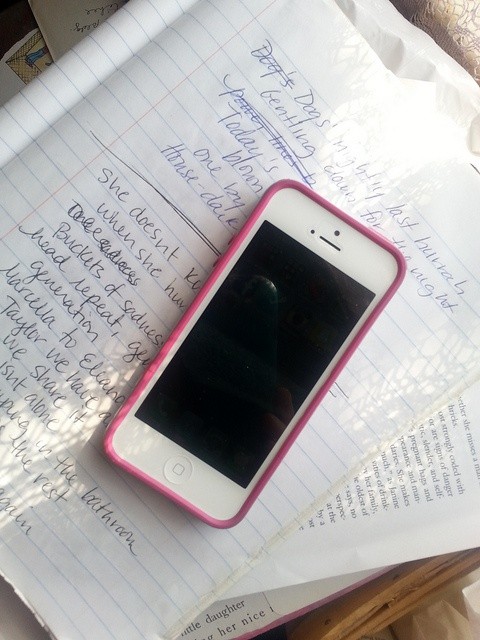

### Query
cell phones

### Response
[{"left": 105, "top": 180, "right": 406, "bottom": 529}]
